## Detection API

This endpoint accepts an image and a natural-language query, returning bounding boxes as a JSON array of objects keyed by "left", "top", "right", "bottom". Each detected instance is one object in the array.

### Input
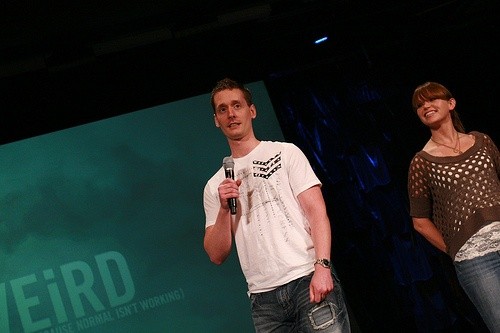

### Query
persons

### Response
[
  {"left": 409, "top": 82, "right": 500, "bottom": 333},
  {"left": 203, "top": 77, "right": 352, "bottom": 333}
]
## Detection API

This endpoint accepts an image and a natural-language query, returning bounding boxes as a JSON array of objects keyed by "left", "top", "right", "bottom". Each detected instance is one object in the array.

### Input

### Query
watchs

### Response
[{"left": 312, "top": 258, "right": 331, "bottom": 270}]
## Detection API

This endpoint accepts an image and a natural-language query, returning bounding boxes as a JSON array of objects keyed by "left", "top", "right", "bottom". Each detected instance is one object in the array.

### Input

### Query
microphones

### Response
[{"left": 223, "top": 157, "right": 238, "bottom": 215}]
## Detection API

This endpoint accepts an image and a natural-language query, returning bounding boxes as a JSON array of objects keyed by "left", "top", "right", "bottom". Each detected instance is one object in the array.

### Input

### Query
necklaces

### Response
[{"left": 430, "top": 131, "right": 459, "bottom": 155}]
